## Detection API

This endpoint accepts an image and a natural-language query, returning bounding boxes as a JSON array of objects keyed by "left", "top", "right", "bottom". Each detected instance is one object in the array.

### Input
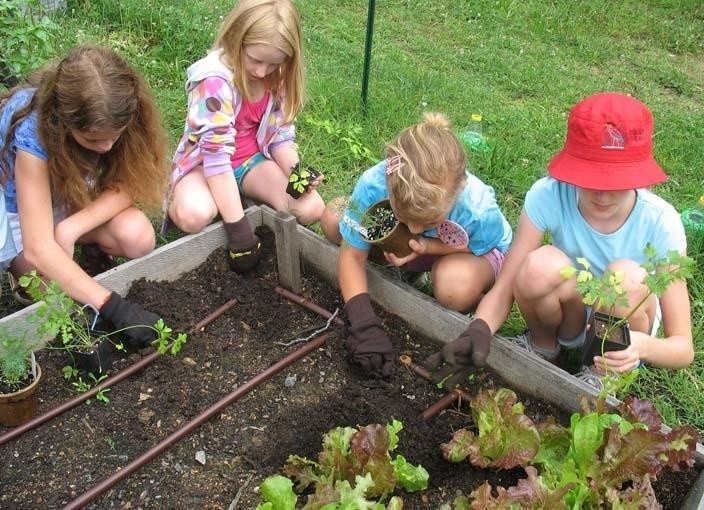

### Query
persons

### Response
[
  {"left": 423, "top": 89, "right": 694, "bottom": 399},
  {"left": 0, "top": 41, "right": 167, "bottom": 349},
  {"left": 320, "top": 112, "right": 511, "bottom": 379},
  {"left": 158, "top": 0, "right": 327, "bottom": 275}
]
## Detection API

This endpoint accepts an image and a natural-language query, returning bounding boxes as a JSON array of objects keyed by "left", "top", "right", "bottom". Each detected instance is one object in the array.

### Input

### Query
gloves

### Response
[
  {"left": 345, "top": 292, "right": 395, "bottom": 379},
  {"left": 425, "top": 318, "right": 492, "bottom": 392},
  {"left": 97, "top": 291, "right": 168, "bottom": 353},
  {"left": 228, "top": 215, "right": 261, "bottom": 273}
]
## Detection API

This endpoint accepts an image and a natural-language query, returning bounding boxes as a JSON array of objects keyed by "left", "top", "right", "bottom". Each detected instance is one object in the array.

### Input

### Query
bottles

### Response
[
  {"left": 689, "top": 194, "right": 703, "bottom": 223},
  {"left": 464, "top": 114, "right": 482, "bottom": 146}
]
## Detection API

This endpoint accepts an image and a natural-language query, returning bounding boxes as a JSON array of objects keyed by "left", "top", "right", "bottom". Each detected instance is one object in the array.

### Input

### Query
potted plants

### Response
[
  {"left": 280, "top": 109, "right": 376, "bottom": 199},
  {"left": 0, "top": 327, "right": 45, "bottom": 429},
  {"left": 340, "top": 190, "right": 420, "bottom": 259},
  {"left": 17, "top": 268, "right": 181, "bottom": 399},
  {"left": 561, "top": 239, "right": 697, "bottom": 367}
]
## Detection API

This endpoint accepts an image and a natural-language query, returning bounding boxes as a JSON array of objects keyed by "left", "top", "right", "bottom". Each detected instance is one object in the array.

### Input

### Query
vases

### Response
[{"left": 228, "top": 241, "right": 263, "bottom": 275}]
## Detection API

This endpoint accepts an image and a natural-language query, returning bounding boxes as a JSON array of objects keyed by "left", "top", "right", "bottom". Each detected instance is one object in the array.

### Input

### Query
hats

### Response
[{"left": 548, "top": 92, "right": 668, "bottom": 190}]
